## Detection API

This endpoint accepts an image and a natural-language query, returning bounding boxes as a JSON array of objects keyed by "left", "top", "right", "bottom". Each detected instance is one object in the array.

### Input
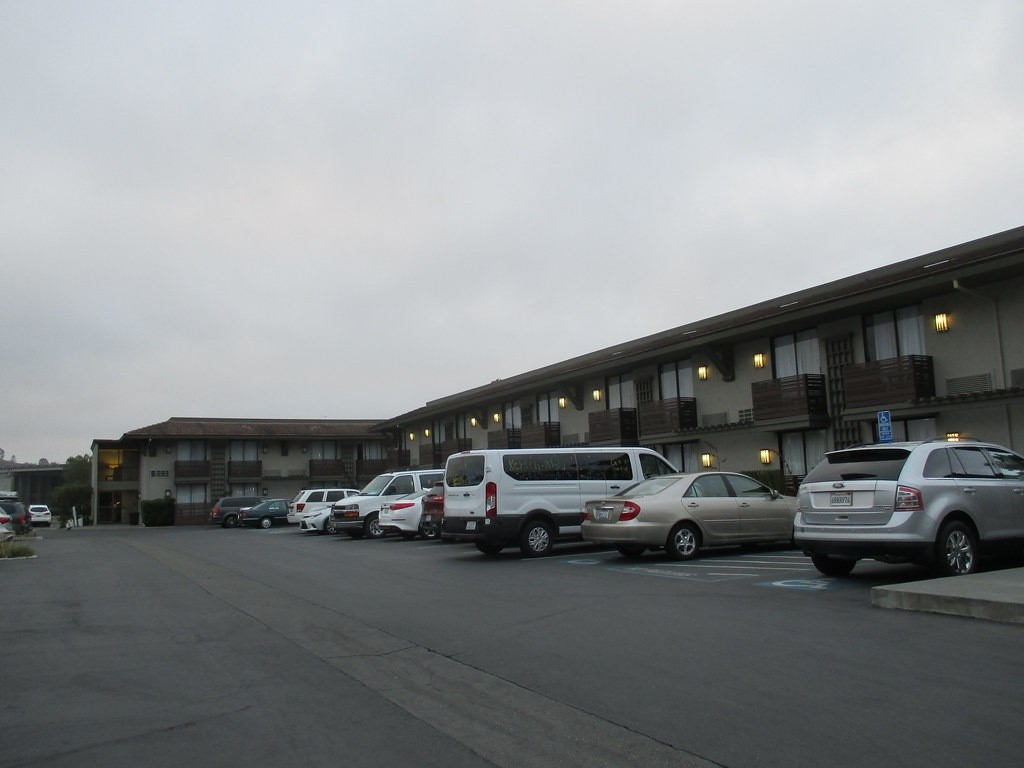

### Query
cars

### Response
[
  {"left": 580, "top": 469, "right": 800, "bottom": 560},
  {"left": 240, "top": 499, "right": 290, "bottom": 528},
  {"left": 378, "top": 489, "right": 425, "bottom": 537},
  {"left": 301, "top": 507, "right": 337, "bottom": 536}
]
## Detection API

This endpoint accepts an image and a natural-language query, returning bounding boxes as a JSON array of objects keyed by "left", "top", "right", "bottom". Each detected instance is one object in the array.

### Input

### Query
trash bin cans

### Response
[{"left": 129, "top": 511, "right": 140, "bottom": 525}]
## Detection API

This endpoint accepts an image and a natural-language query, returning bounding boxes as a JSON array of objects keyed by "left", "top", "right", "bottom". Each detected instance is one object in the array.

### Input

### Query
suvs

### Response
[
  {"left": 211, "top": 496, "right": 271, "bottom": 527},
  {"left": 0, "top": 506, "right": 15, "bottom": 541},
  {"left": 27, "top": 505, "right": 51, "bottom": 525},
  {"left": 786, "top": 436, "right": 1024, "bottom": 579},
  {"left": 418, "top": 483, "right": 451, "bottom": 539},
  {"left": 1, "top": 490, "right": 30, "bottom": 532}
]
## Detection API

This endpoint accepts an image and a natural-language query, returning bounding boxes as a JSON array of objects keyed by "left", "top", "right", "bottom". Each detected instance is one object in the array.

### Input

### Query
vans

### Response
[
  {"left": 287, "top": 489, "right": 360, "bottom": 525},
  {"left": 331, "top": 470, "right": 445, "bottom": 538},
  {"left": 439, "top": 445, "right": 683, "bottom": 553}
]
